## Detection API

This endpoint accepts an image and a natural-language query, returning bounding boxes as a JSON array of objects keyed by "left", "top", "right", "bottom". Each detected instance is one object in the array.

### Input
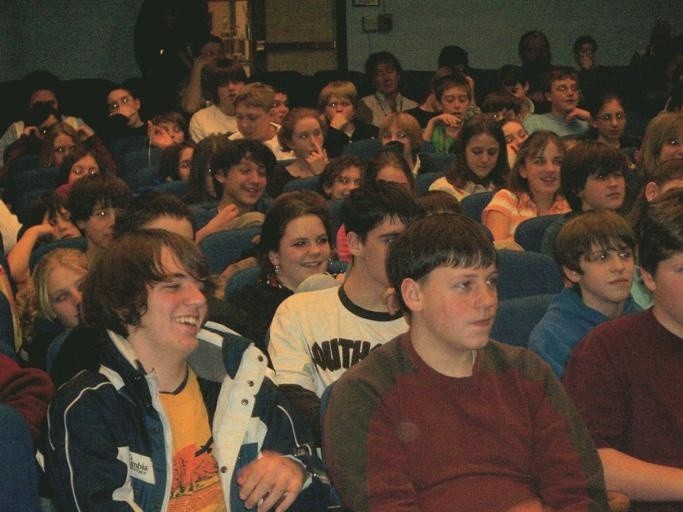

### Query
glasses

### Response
[{"left": 594, "top": 111, "right": 626, "bottom": 121}]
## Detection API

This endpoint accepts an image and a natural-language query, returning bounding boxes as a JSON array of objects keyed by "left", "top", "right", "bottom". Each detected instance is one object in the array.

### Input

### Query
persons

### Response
[
  {"left": 1, "top": 3, "right": 681, "bottom": 432},
  {"left": 41, "top": 229, "right": 323, "bottom": 511},
  {"left": 319, "top": 210, "right": 606, "bottom": 511},
  {"left": 262, "top": 184, "right": 423, "bottom": 449},
  {"left": 0, "top": 405, "right": 41, "bottom": 511},
  {"left": 561, "top": 189, "right": 683, "bottom": 508}
]
